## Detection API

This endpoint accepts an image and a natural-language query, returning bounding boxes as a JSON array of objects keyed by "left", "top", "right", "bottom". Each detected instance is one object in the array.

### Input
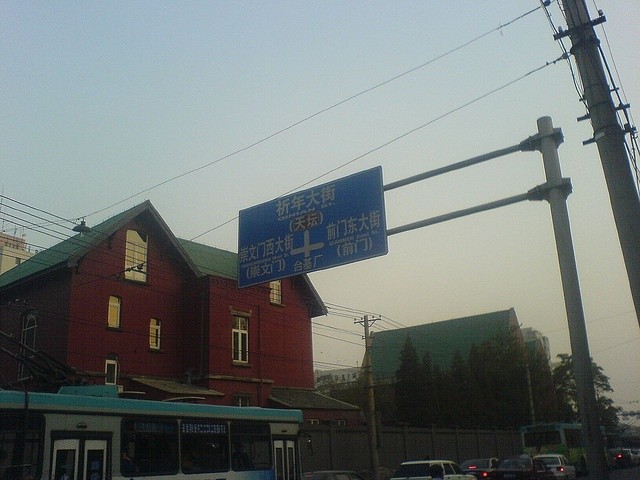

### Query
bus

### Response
[
  {"left": 521, "top": 423, "right": 613, "bottom": 477},
  {"left": 0, "top": 390, "right": 314, "bottom": 480}
]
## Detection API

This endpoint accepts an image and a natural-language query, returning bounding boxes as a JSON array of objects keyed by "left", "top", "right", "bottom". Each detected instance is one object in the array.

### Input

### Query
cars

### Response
[
  {"left": 390, "top": 459, "right": 478, "bottom": 480},
  {"left": 461, "top": 458, "right": 499, "bottom": 480},
  {"left": 485, "top": 457, "right": 557, "bottom": 480},
  {"left": 534, "top": 454, "right": 576, "bottom": 480},
  {"left": 303, "top": 471, "right": 367, "bottom": 480}
]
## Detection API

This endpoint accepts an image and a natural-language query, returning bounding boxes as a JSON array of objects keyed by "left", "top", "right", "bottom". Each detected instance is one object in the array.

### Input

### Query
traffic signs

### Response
[{"left": 238, "top": 165, "right": 388, "bottom": 289}]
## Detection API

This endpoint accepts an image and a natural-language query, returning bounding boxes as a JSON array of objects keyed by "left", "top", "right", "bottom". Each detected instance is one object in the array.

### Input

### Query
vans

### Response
[{"left": 622, "top": 448, "right": 640, "bottom": 465}]
